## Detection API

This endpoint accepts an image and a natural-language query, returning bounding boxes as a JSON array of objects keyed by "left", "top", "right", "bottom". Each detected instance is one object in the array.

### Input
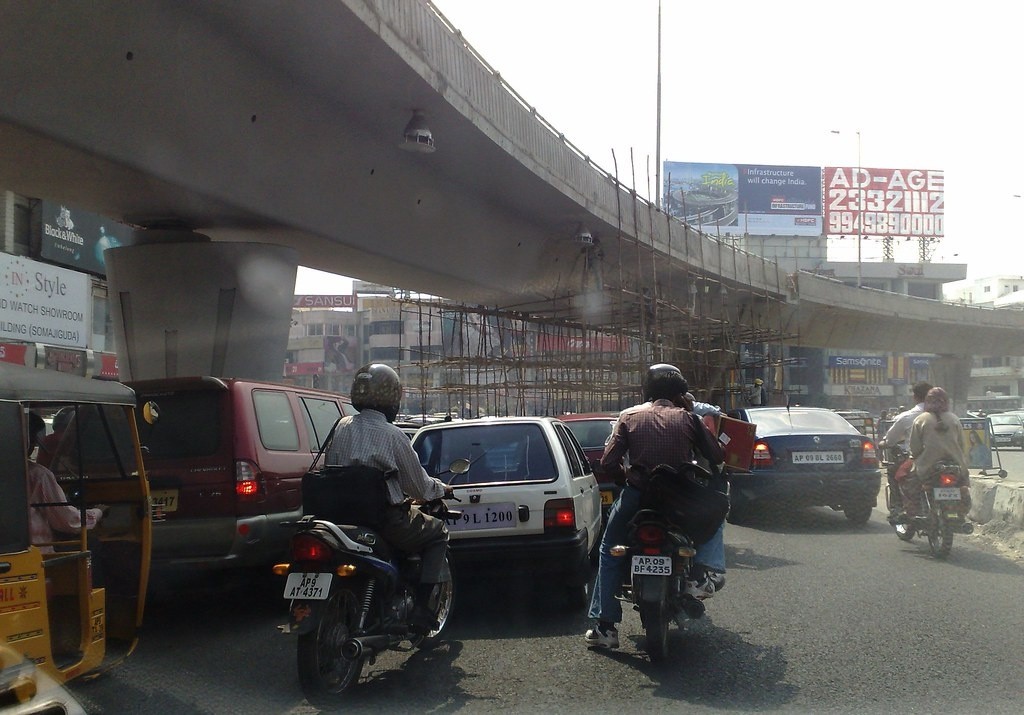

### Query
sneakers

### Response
[
  {"left": 683, "top": 574, "right": 715, "bottom": 602},
  {"left": 584, "top": 623, "right": 620, "bottom": 649}
]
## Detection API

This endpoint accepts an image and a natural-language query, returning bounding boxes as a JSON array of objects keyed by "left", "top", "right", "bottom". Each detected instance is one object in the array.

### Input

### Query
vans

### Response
[{"left": 31, "top": 374, "right": 364, "bottom": 598}]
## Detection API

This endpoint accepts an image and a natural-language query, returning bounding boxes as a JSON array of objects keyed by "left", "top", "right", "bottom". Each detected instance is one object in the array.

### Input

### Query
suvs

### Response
[{"left": 558, "top": 414, "right": 626, "bottom": 514}]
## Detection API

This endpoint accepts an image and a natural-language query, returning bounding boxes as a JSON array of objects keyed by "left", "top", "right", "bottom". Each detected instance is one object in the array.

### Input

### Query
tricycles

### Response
[{"left": 0, "top": 359, "right": 160, "bottom": 708}]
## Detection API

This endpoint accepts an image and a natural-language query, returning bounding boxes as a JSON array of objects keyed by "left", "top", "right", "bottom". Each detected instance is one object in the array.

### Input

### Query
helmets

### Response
[
  {"left": 643, "top": 364, "right": 682, "bottom": 402},
  {"left": 351, "top": 362, "right": 405, "bottom": 422},
  {"left": 879, "top": 410, "right": 888, "bottom": 417}
]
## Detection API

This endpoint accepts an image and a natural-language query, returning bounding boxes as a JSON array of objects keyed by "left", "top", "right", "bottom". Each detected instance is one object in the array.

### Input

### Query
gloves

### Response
[{"left": 444, "top": 483, "right": 454, "bottom": 500}]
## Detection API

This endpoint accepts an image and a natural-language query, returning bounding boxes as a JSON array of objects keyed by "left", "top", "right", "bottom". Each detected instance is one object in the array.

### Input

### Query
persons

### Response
[
  {"left": 748, "top": 378, "right": 767, "bottom": 407},
  {"left": 584, "top": 363, "right": 726, "bottom": 647},
  {"left": 26, "top": 406, "right": 107, "bottom": 553},
  {"left": 323, "top": 363, "right": 455, "bottom": 629},
  {"left": 968, "top": 431, "right": 990, "bottom": 467},
  {"left": 976, "top": 422, "right": 984, "bottom": 430},
  {"left": 879, "top": 381, "right": 971, "bottom": 522}
]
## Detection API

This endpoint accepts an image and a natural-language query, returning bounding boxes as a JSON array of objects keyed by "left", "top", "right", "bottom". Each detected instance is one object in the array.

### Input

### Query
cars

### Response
[
  {"left": 984, "top": 413, "right": 1024, "bottom": 450},
  {"left": 724, "top": 404, "right": 885, "bottom": 525},
  {"left": 410, "top": 413, "right": 605, "bottom": 574}
]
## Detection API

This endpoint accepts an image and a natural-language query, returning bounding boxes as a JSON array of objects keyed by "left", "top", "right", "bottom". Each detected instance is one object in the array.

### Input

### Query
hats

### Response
[
  {"left": 754, "top": 379, "right": 762, "bottom": 385},
  {"left": 924, "top": 387, "right": 948, "bottom": 412}
]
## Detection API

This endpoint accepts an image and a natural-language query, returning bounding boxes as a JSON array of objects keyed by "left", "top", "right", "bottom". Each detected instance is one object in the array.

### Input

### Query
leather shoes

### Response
[{"left": 709, "top": 575, "right": 725, "bottom": 592}]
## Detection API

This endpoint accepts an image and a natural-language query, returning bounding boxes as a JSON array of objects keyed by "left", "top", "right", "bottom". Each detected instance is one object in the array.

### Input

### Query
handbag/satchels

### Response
[
  {"left": 300, "top": 465, "right": 391, "bottom": 531},
  {"left": 646, "top": 463, "right": 730, "bottom": 545}
]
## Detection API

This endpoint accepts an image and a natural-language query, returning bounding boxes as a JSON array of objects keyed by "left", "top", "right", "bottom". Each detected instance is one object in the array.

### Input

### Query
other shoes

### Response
[{"left": 411, "top": 605, "right": 440, "bottom": 631}]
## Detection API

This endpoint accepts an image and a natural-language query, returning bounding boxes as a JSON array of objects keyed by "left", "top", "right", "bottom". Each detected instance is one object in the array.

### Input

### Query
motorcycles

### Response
[
  {"left": 273, "top": 458, "right": 473, "bottom": 711},
  {"left": 590, "top": 433, "right": 731, "bottom": 661},
  {"left": 877, "top": 435, "right": 975, "bottom": 560}
]
